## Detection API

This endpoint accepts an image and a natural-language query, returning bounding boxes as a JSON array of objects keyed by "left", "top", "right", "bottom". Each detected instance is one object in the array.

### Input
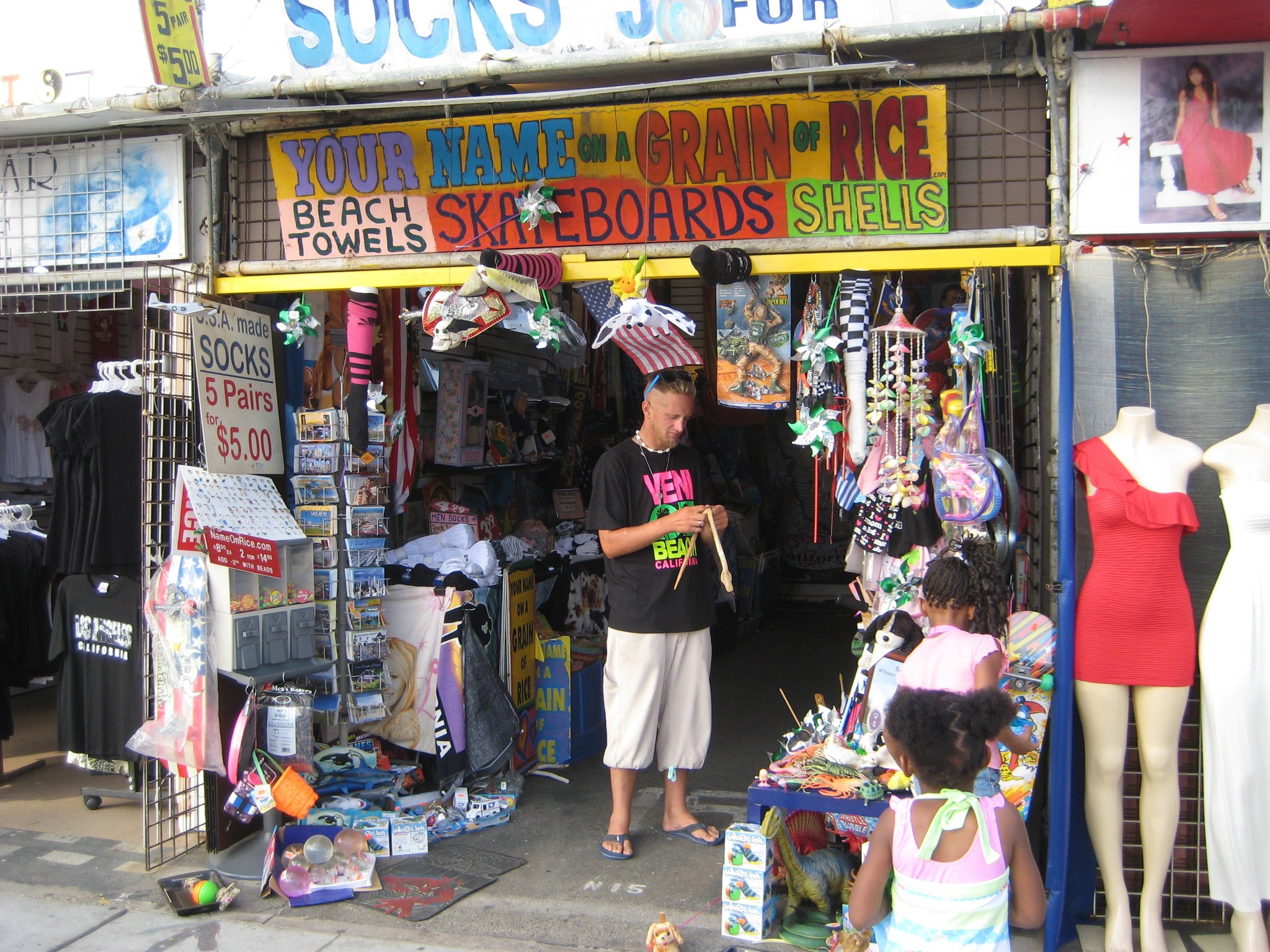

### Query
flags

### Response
[
  {"left": 881, "top": 283, "right": 909, "bottom": 322},
  {"left": 576, "top": 279, "right": 704, "bottom": 375}
]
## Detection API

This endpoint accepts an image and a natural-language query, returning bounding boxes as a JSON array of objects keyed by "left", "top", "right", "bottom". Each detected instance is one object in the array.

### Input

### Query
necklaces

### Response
[{"left": 635, "top": 430, "right": 671, "bottom": 454}]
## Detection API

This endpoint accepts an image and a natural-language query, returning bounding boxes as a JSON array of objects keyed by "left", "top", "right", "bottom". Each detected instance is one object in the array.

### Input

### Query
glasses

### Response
[{"left": 644, "top": 371, "right": 692, "bottom": 401}]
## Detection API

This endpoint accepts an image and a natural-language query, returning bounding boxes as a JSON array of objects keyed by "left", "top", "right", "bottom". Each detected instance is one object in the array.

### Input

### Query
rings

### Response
[
  {"left": 698, "top": 520, "right": 700, "bottom": 527},
  {"left": 695, "top": 528, "right": 698, "bottom": 533}
]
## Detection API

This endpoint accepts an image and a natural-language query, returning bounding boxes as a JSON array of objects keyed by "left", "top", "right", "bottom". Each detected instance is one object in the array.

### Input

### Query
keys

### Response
[{"left": 657, "top": 492, "right": 664, "bottom": 506}]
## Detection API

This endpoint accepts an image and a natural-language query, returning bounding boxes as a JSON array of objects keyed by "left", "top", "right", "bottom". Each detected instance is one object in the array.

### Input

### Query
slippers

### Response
[
  {"left": 601, "top": 832, "right": 633, "bottom": 860},
  {"left": 665, "top": 820, "right": 725, "bottom": 846}
]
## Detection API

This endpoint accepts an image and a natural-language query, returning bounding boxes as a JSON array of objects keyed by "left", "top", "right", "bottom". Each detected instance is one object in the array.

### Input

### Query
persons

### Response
[
  {"left": 1160, "top": 62, "right": 1255, "bottom": 225},
  {"left": 887, "top": 529, "right": 1040, "bottom": 801},
  {"left": 356, "top": 636, "right": 421, "bottom": 749},
  {"left": 1074, "top": 404, "right": 1204, "bottom": 952},
  {"left": 1199, "top": 401, "right": 1270, "bottom": 952},
  {"left": 839, "top": 269, "right": 872, "bottom": 466},
  {"left": 513, "top": 392, "right": 558, "bottom": 453},
  {"left": 902, "top": 280, "right": 921, "bottom": 325},
  {"left": 587, "top": 369, "right": 727, "bottom": 859},
  {"left": 850, "top": 683, "right": 1046, "bottom": 952},
  {"left": 729, "top": 290, "right": 785, "bottom": 393},
  {"left": 345, "top": 285, "right": 380, "bottom": 451},
  {"left": 938, "top": 283, "right": 964, "bottom": 308},
  {"left": 493, "top": 421, "right": 516, "bottom": 456}
]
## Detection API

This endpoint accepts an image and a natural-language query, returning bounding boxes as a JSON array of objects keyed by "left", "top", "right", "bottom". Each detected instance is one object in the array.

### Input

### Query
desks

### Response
[{"left": 368, "top": 552, "right": 602, "bottom": 796}]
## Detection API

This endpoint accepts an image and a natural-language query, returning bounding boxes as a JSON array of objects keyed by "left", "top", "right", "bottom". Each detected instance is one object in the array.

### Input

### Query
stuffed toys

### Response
[
  {"left": 646, "top": 911, "right": 683, "bottom": 952},
  {"left": 858, "top": 610, "right": 923, "bottom": 668},
  {"left": 608, "top": 254, "right": 648, "bottom": 301}
]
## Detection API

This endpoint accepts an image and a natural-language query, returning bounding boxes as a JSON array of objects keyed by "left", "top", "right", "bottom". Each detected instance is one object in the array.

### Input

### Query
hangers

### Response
[
  {"left": 0, "top": 502, "right": 44, "bottom": 539},
  {"left": 87, "top": 361, "right": 157, "bottom": 395},
  {"left": 0, "top": 354, "right": 59, "bottom": 390}
]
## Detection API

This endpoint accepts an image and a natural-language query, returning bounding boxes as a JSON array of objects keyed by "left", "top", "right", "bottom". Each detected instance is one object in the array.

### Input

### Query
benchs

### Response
[{"left": 1148, "top": 132, "right": 1262, "bottom": 202}]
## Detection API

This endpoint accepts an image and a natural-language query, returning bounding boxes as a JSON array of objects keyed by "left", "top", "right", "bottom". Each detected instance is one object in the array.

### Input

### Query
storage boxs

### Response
[
  {"left": 721, "top": 822, "right": 775, "bottom": 941},
  {"left": 303, "top": 729, "right": 519, "bottom": 861}
]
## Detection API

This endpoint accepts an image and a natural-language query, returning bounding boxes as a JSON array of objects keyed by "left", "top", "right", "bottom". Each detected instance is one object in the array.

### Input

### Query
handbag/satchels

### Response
[{"left": 932, "top": 387, "right": 1002, "bottom": 525}]
